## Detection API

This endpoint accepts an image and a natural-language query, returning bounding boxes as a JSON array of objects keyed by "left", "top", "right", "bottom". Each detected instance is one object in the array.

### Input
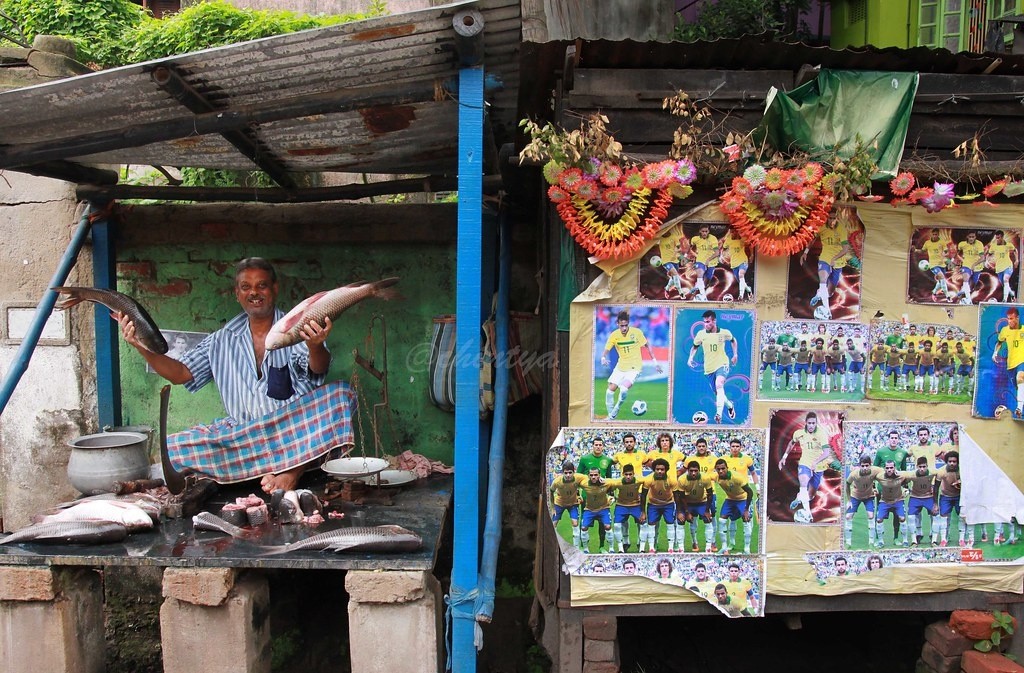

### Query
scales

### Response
[{"left": 321, "top": 312, "right": 419, "bottom": 488}]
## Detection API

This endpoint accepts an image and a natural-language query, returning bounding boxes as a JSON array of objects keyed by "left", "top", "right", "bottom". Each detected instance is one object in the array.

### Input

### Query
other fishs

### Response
[
  {"left": 254, "top": 525, "right": 423, "bottom": 557},
  {"left": 50, "top": 287, "right": 169, "bottom": 355},
  {"left": 0, "top": 479, "right": 344, "bottom": 544},
  {"left": 265, "top": 277, "right": 400, "bottom": 351}
]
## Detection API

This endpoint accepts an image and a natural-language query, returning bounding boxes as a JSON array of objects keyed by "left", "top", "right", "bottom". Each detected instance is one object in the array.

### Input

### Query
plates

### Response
[
  {"left": 320, "top": 456, "right": 390, "bottom": 477},
  {"left": 360, "top": 470, "right": 416, "bottom": 485}
]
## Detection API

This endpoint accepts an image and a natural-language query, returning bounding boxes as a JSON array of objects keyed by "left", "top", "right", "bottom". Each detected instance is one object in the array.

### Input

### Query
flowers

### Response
[{"left": 543, "top": 162, "right": 1009, "bottom": 210}]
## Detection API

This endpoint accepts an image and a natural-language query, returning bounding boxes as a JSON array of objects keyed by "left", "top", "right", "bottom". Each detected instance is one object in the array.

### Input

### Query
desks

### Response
[{"left": 0, "top": 469, "right": 452, "bottom": 673}]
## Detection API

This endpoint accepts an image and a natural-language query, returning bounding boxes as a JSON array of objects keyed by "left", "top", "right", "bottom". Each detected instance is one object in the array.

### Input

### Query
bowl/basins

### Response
[{"left": 67, "top": 432, "right": 149, "bottom": 495}]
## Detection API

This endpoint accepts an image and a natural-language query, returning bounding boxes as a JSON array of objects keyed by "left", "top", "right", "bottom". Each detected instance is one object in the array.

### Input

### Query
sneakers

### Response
[
  {"left": 689, "top": 287, "right": 699, "bottom": 294},
  {"left": 738, "top": 296, "right": 744, "bottom": 303},
  {"left": 680, "top": 293, "right": 686, "bottom": 299},
  {"left": 868, "top": 385, "right": 974, "bottom": 398},
  {"left": 704, "top": 297, "right": 708, "bottom": 301},
  {"left": 946, "top": 297, "right": 953, "bottom": 302},
  {"left": 1011, "top": 291, "right": 1018, "bottom": 303},
  {"left": 790, "top": 499, "right": 800, "bottom": 510},
  {"left": 810, "top": 295, "right": 821, "bottom": 306},
  {"left": 728, "top": 401, "right": 735, "bottom": 419},
  {"left": 758, "top": 384, "right": 865, "bottom": 394},
  {"left": 932, "top": 292, "right": 938, "bottom": 303},
  {"left": 847, "top": 533, "right": 1021, "bottom": 548},
  {"left": 609, "top": 413, "right": 617, "bottom": 419},
  {"left": 665, "top": 288, "right": 670, "bottom": 299},
  {"left": 715, "top": 414, "right": 722, "bottom": 424},
  {"left": 826, "top": 308, "right": 832, "bottom": 320},
  {"left": 747, "top": 287, "right": 753, "bottom": 300},
  {"left": 1015, "top": 408, "right": 1022, "bottom": 419},
  {"left": 809, "top": 514, "right": 813, "bottom": 523},
  {"left": 575, "top": 543, "right": 751, "bottom": 554}
]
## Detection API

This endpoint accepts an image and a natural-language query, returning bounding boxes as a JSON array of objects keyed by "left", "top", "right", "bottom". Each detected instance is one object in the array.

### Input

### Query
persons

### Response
[
  {"left": 779, "top": 412, "right": 830, "bottom": 522},
  {"left": 758, "top": 323, "right": 975, "bottom": 395},
  {"left": 951, "top": 230, "right": 986, "bottom": 304},
  {"left": 602, "top": 308, "right": 664, "bottom": 422},
  {"left": 800, "top": 207, "right": 850, "bottom": 319},
  {"left": 657, "top": 225, "right": 753, "bottom": 302},
  {"left": 991, "top": 308, "right": 1024, "bottom": 419},
  {"left": 547, "top": 430, "right": 760, "bottom": 617},
  {"left": 688, "top": 310, "right": 737, "bottom": 423},
  {"left": 868, "top": 555, "right": 882, "bottom": 570},
  {"left": 844, "top": 426, "right": 1021, "bottom": 550},
  {"left": 118, "top": 256, "right": 359, "bottom": 496},
  {"left": 984, "top": 230, "right": 1019, "bottom": 303},
  {"left": 835, "top": 557, "right": 849, "bottom": 576},
  {"left": 911, "top": 228, "right": 953, "bottom": 303}
]
